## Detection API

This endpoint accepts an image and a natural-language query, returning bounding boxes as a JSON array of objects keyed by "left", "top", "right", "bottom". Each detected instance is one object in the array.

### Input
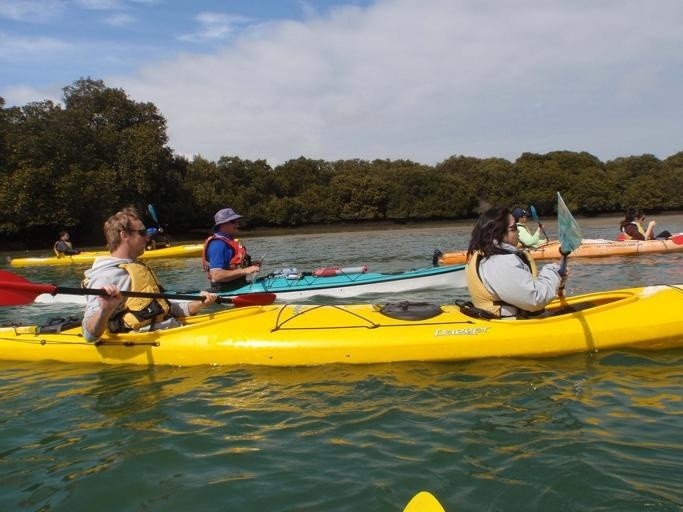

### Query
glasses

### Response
[
  {"left": 509, "top": 222, "right": 518, "bottom": 230},
  {"left": 120, "top": 229, "right": 147, "bottom": 236}
]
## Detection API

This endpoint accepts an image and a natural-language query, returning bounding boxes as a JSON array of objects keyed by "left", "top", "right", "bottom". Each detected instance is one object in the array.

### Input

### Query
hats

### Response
[
  {"left": 214, "top": 209, "right": 244, "bottom": 227},
  {"left": 513, "top": 208, "right": 529, "bottom": 218}
]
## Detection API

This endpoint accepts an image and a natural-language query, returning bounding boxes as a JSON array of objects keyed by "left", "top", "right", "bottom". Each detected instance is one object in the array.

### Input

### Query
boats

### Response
[
  {"left": 0, "top": 279, "right": 681, "bottom": 367},
  {"left": 432, "top": 230, "right": 682, "bottom": 268},
  {"left": 9, "top": 238, "right": 239, "bottom": 269},
  {"left": 34, "top": 261, "right": 469, "bottom": 314}
]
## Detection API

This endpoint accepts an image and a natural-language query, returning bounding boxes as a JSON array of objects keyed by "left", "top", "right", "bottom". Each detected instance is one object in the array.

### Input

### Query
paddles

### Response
[
  {"left": 248, "top": 249, "right": 267, "bottom": 292},
  {"left": 557, "top": 191, "right": 583, "bottom": 300},
  {"left": 147, "top": 204, "right": 165, "bottom": 234},
  {"left": 0, "top": 269, "right": 276, "bottom": 308},
  {"left": 530, "top": 205, "right": 550, "bottom": 243}
]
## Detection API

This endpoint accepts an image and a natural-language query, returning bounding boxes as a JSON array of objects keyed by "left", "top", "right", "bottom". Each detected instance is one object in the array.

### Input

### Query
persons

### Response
[
  {"left": 81, "top": 208, "right": 218, "bottom": 342},
  {"left": 145, "top": 228, "right": 168, "bottom": 250},
  {"left": 621, "top": 208, "right": 656, "bottom": 241},
  {"left": 512, "top": 207, "right": 548, "bottom": 252},
  {"left": 54, "top": 231, "right": 80, "bottom": 257},
  {"left": 202, "top": 208, "right": 261, "bottom": 291},
  {"left": 466, "top": 205, "right": 568, "bottom": 320}
]
